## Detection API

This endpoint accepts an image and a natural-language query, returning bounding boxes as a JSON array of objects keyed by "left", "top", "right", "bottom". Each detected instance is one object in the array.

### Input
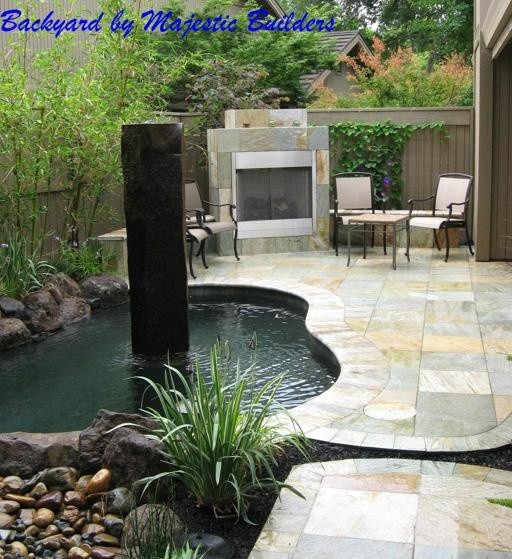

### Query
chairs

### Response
[
  {"left": 182, "top": 179, "right": 240, "bottom": 279},
  {"left": 330, "top": 172, "right": 474, "bottom": 271}
]
